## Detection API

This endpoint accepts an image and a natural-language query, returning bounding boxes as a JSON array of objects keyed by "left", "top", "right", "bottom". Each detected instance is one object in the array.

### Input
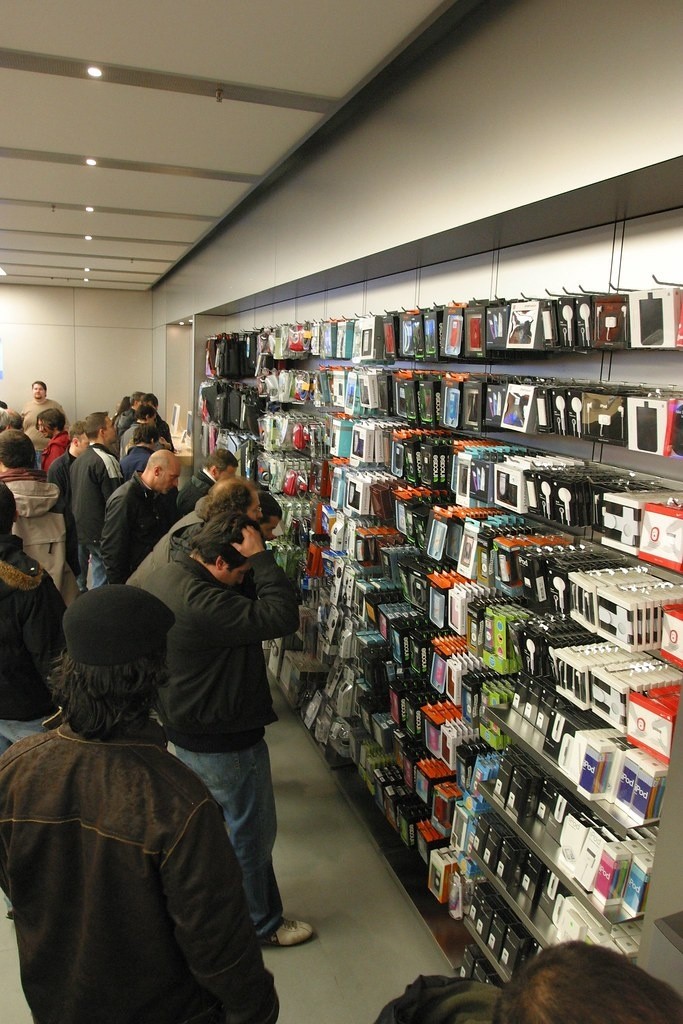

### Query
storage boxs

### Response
[{"left": 263, "top": 285, "right": 683, "bottom": 992}]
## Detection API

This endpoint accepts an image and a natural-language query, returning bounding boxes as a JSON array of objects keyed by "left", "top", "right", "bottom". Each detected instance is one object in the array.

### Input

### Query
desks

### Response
[{"left": 171, "top": 429, "right": 192, "bottom": 491}]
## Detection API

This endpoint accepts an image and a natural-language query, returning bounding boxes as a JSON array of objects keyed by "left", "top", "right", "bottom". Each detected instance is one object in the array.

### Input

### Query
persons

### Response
[
  {"left": 36, "top": 408, "right": 70, "bottom": 481},
  {"left": 0, "top": 400, "right": 22, "bottom": 433},
  {"left": 176, "top": 449, "right": 238, "bottom": 515},
  {"left": 100, "top": 449, "right": 180, "bottom": 584},
  {"left": 111, "top": 391, "right": 174, "bottom": 459},
  {"left": 46, "top": 421, "right": 89, "bottom": 577},
  {"left": 0, "top": 429, "right": 66, "bottom": 590},
  {"left": 119, "top": 424, "right": 160, "bottom": 481},
  {"left": 257, "top": 492, "right": 282, "bottom": 540},
  {"left": 69, "top": 412, "right": 125, "bottom": 593},
  {"left": 370, "top": 939, "right": 683, "bottom": 1024},
  {"left": 148, "top": 510, "right": 313, "bottom": 948},
  {"left": 0, "top": 585, "right": 280, "bottom": 1024},
  {"left": 0, "top": 482, "right": 68, "bottom": 919},
  {"left": 20, "top": 381, "right": 69, "bottom": 471},
  {"left": 125, "top": 477, "right": 263, "bottom": 615}
]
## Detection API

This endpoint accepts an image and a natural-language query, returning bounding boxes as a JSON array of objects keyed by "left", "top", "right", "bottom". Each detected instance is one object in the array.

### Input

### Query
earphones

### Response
[
  {"left": 549, "top": 646, "right": 559, "bottom": 681},
  {"left": 621, "top": 306, "right": 627, "bottom": 318},
  {"left": 571, "top": 397, "right": 582, "bottom": 433},
  {"left": 562, "top": 304, "right": 591, "bottom": 347},
  {"left": 587, "top": 402, "right": 593, "bottom": 414},
  {"left": 556, "top": 395, "right": 566, "bottom": 432},
  {"left": 558, "top": 661, "right": 563, "bottom": 673},
  {"left": 618, "top": 406, "right": 624, "bottom": 418},
  {"left": 596, "top": 306, "right": 604, "bottom": 318},
  {"left": 541, "top": 482, "right": 571, "bottom": 527},
  {"left": 552, "top": 577, "right": 590, "bottom": 614},
  {"left": 575, "top": 671, "right": 582, "bottom": 685},
  {"left": 526, "top": 639, "right": 535, "bottom": 676}
]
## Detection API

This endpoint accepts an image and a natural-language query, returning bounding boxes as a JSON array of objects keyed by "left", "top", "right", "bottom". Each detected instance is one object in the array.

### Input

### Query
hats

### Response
[{"left": 62, "top": 584, "right": 175, "bottom": 666}]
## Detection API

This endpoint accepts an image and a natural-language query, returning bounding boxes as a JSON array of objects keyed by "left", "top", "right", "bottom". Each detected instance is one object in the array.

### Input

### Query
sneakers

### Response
[{"left": 256, "top": 917, "right": 313, "bottom": 946}]
